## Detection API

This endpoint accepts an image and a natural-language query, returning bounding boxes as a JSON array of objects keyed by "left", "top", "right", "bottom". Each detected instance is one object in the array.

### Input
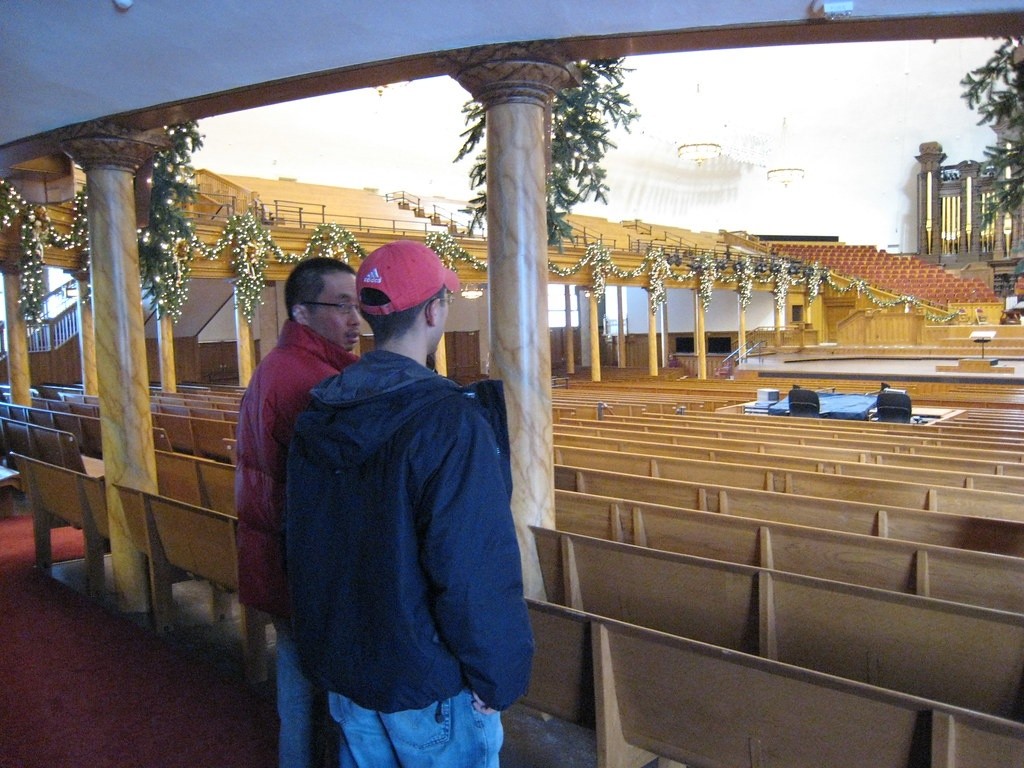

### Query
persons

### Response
[
  {"left": 236, "top": 257, "right": 362, "bottom": 768},
  {"left": 286, "top": 241, "right": 534, "bottom": 768}
]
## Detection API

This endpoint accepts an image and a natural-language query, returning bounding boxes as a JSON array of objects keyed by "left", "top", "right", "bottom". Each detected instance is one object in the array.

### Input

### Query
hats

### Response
[{"left": 355, "top": 240, "right": 457, "bottom": 316}]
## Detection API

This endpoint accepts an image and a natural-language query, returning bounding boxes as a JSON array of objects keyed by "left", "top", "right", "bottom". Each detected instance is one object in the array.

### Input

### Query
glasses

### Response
[{"left": 300, "top": 301, "right": 360, "bottom": 314}]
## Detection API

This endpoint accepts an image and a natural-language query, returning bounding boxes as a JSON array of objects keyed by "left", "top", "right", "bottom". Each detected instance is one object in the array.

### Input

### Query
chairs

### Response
[
  {"left": 958, "top": 308, "right": 971, "bottom": 325},
  {"left": 975, "top": 308, "right": 988, "bottom": 325},
  {"left": 759, "top": 243, "right": 1004, "bottom": 311},
  {"left": 785, "top": 389, "right": 831, "bottom": 418},
  {"left": 871, "top": 393, "right": 922, "bottom": 425}
]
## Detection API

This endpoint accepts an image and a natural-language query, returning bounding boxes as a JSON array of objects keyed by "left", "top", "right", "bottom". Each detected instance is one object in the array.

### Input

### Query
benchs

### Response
[
  {"left": 217, "top": 174, "right": 747, "bottom": 260},
  {"left": 0, "top": 364, "right": 1024, "bottom": 768}
]
